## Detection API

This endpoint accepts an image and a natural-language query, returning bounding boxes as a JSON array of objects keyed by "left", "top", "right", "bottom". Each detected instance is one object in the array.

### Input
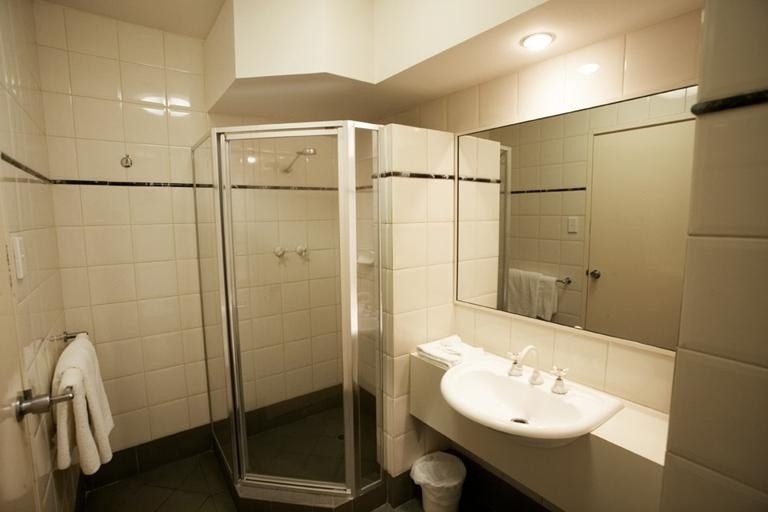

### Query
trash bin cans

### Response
[{"left": 412, "top": 451, "right": 466, "bottom": 512}]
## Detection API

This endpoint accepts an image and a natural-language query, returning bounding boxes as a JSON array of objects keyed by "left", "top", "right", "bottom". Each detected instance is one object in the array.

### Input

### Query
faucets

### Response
[{"left": 515, "top": 345, "right": 542, "bottom": 382}]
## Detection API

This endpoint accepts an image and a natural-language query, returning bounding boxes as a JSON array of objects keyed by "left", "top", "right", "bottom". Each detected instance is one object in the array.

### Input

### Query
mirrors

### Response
[{"left": 452, "top": 83, "right": 697, "bottom": 357}]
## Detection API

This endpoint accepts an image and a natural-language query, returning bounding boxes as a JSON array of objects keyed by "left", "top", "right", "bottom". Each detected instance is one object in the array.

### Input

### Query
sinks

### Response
[{"left": 442, "top": 355, "right": 626, "bottom": 449}]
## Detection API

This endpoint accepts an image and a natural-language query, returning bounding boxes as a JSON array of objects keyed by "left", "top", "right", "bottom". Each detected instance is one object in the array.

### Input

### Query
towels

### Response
[
  {"left": 52, "top": 334, "right": 113, "bottom": 476},
  {"left": 503, "top": 268, "right": 558, "bottom": 321}
]
